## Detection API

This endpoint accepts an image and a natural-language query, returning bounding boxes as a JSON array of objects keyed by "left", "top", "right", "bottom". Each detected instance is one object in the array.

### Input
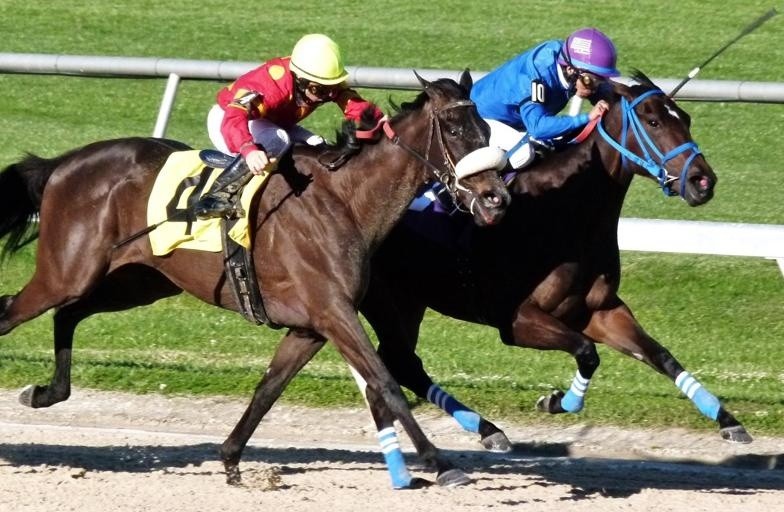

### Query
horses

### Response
[
  {"left": 357, "top": 66, "right": 754, "bottom": 489},
  {"left": 0, "top": 66, "right": 513, "bottom": 488}
]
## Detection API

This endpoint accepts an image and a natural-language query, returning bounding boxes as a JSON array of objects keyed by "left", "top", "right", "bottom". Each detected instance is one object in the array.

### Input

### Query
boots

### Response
[{"left": 193, "top": 152, "right": 250, "bottom": 219}]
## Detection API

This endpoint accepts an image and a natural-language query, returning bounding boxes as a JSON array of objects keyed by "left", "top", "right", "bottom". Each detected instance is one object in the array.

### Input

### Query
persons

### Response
[
  {"left": 426, "top": 27, "right": 622, "bottom": 216},
  {"left": 194, "top": 31, "right": 383, "bottom": 219}
]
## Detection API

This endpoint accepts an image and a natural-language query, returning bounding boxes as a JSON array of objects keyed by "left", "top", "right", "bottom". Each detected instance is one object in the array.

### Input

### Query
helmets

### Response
[
  {"left": 289, "top": 33, "right": 351, "bottom": 87},
  {"left": 558, "top": 27, "right": 622, "bottom": 78}
]
